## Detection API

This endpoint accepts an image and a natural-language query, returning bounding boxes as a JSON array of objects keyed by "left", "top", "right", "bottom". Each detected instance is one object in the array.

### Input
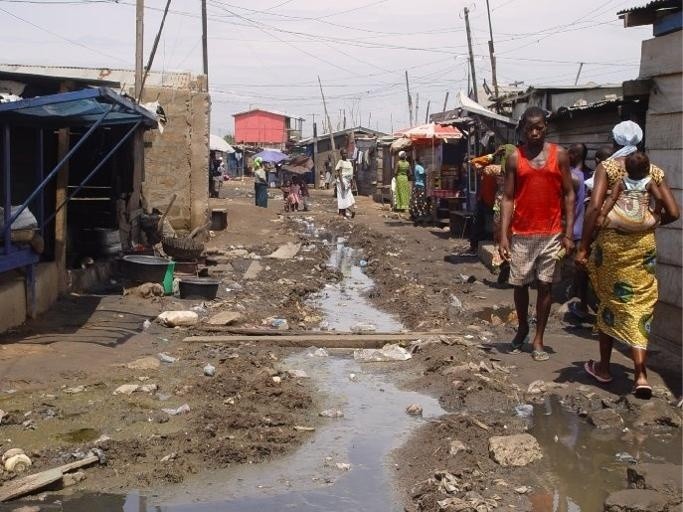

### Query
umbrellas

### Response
[
  {"left": 210, "top": 134, "right": 236, "bottom": 154},
  {"left": 251, "top": 150, "right": 289, "bottom": 165},
  {"left": 393, "top": 122, "right": 464, "bottom": 172}
]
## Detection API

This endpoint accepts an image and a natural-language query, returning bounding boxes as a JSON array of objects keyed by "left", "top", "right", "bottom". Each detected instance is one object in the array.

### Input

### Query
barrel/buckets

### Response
[{"left": 211, "top": 208, "right": 227, "bottom": 230}]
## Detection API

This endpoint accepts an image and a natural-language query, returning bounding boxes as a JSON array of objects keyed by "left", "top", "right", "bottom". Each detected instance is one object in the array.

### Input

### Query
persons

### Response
[
  {"left": 582, "top": 121, "right": 679, "bottom": 396},
  {"left": 281, "top": 169, "right": 330, "bottom": 213},
  {"left": 334, "top": 148, "right": 355, "bottom": 220},
  {"left": 499, "top": 109, "right": 574, "bottom": 361},
  {"left": 469, "top": 145, "right": 518, "bottom": 286},
  {"left": 568, "top": 146, "right": 612, "bottom": 318},
  {"left": 566, "top": 143, "right": 595, "bottom": 242},
  {"left": 409, "top": 161, "right": 428, "bottom": 218},
  {"left": 393, "top": 151, "right": 413, "bottom": 211},
  {"left": 253, "top": 157, "right": 268, "bottom": 208},
  {"left": 596, "top": 151, "right": 663, "bottom": 233},
  {"left": 217, "top": 156, "right": 225, "bottom": 188}
]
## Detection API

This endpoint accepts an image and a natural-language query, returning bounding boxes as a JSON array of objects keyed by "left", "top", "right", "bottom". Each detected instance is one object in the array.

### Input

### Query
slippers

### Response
[
  {"left": 531, "top": 350, "right": 550, "bottom": 361},
  {"left": 506, "top": 342, "right": 523, "bottom": 356},
  {"left": 632, "top": 382, "right": 654, "bottom": 397},
  {"left": 585, "top": 360, "right": 613, "bottom": 384}
]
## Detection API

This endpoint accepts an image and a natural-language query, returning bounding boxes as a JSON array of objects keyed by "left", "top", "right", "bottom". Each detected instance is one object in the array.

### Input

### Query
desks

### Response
[{"left": 431, "top": 190, "right": 467, "bottom": 222}]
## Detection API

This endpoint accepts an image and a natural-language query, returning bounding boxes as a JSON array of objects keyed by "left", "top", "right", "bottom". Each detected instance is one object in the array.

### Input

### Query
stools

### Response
[{"left": 449, "top": 210, "right": 474, "bottom": 240}]
